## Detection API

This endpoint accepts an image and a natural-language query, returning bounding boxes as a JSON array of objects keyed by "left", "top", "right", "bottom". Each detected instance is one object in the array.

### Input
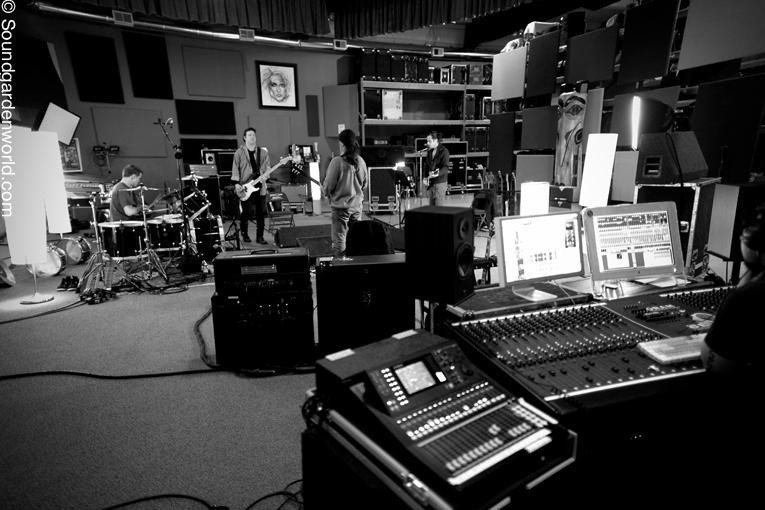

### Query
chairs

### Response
[{"left": 265, "top": 192, "right": 296, "bottom": 233}]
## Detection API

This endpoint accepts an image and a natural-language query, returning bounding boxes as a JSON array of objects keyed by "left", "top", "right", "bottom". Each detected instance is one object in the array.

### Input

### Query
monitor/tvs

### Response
[
  {"left": 494, "top": 211, "right": 585, "bottom": 302},
  {"left": 583, "top": 201, "right": 684, "bottom": 289}
]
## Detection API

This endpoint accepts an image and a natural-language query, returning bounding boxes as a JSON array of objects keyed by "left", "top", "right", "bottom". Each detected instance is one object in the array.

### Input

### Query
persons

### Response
[
  {"left": 320, "top": 128, "right": 370, "bottom": 259},
  {"left": 424, "top": 130, "right": 451, "bottom": 207},
  {"left": 698, "top": 278, "right": 765, "bottom": 378},
  {"left": 231, "top": 127, "right": 271, "bottom": 245},
  {"left": 108, "top": 163, "right": 146, "bottom": 221},
  {"left": 260, "top": 66, "right": 294, "bottom": 105}
]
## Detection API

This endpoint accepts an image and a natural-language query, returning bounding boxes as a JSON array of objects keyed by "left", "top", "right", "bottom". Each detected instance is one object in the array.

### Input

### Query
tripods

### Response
[
  {"left": 155, "top": 123, "right": 212, "bottom": 279},
  {"left": 73, "top": 199, "right": 145, "bottom": 294},
  {"left": 130, "top": 195, "right": 171, "bottom": 284}
]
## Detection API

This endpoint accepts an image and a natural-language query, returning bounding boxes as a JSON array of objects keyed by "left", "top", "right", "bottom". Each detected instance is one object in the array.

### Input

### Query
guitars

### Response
[{"left": 236, "top": 153, "right": 293, "bottom": 202}]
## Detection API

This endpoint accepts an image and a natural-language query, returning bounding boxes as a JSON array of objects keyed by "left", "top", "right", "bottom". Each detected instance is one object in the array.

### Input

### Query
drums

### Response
[
  {"left": 146, "top": 214, "right": 185, "bottom": 249},
  {"left": 175, "top": 187, "right": 212, "bottom": 222},
  {"left": 98, "top": 220, "right": 146, "bottom": 257},
  {"left": 186, "top": 214, "right": 226, "bottom": 274}
]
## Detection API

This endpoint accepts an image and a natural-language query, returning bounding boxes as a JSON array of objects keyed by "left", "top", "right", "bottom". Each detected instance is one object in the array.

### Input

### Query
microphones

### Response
[
  {"left": 170, "top": 118, "right": 174, "bottom": 128},
  {"left": 420, "top": 148, "right": 428, "bottom": 152}
]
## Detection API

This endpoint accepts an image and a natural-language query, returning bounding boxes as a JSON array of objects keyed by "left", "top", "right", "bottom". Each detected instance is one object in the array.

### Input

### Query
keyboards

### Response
[{"left": 637, "top": 332, "right": 708, "bottom": 365}]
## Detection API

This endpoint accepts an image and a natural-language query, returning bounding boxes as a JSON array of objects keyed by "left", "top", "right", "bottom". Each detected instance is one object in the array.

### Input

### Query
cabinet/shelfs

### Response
[
  {"left": 323, "top": 79, "right": 465, "bottom": 195},
  {"left": 465, "top": 85, "right": 495, "bottom": 189}
]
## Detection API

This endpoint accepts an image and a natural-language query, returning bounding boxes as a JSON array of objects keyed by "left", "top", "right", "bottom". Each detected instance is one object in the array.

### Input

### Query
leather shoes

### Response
[
  {"left": 256, "top": 238, "right": 267, "bottom": 244},
  {"left": 243, "top": 235, "right": 251, "bottom": 242}
]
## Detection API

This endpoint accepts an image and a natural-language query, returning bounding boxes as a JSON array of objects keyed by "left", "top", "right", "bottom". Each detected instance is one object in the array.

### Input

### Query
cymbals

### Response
[
  {"left": 175, "top": 174, "right": 209, "bottom": 180},
  {"left": 158, "top": 185, "right": 189, "bottom": 201},
  {"left": 118, "top": 186, "right": 160, "bottom": 191}
]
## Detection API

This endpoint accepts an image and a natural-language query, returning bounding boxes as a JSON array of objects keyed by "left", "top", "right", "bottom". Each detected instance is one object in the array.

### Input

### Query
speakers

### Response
[
  {"left": 405, "top": 205, "right": 476, "bottom": 306},
  {"left": 315, "top": 255, "right": 415, "bottom": 359},
  {"left": 635, "top": 131, "right": 708, "bottom": 183},
  {"left": 211, "top": 248, "right": 314, "bottom": 371},
  {"left": 346, "top": 220, "right": 396, "bottom": 254},
  {"left": 273, "top": 224, "right": 332, "bottom": 248}
]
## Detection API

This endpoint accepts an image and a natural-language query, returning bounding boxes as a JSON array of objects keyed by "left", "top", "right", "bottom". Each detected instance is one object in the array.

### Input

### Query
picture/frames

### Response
[{"left": 255, "top": 60, "right": 301, "bottom": 111}]
